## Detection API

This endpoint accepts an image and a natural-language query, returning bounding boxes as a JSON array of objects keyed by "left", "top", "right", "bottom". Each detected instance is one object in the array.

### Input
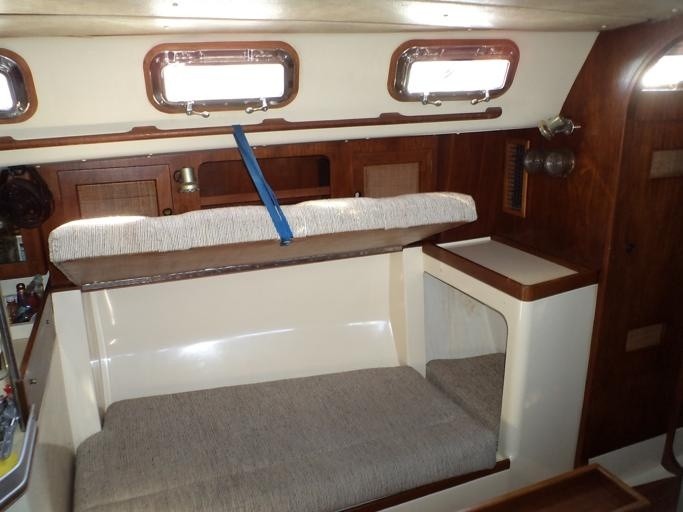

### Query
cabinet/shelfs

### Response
[
  {"left": 342, "top": 133, "right": 439, "bottom": 197},
  {"left": 57, "top": 154, "right": 173, "bottom": 223}
]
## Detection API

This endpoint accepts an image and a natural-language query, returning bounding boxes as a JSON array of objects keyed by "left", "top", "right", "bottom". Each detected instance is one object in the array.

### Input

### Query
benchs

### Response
[{"left": 44, "top": 188, "right": 600, "bottom": 511}]
